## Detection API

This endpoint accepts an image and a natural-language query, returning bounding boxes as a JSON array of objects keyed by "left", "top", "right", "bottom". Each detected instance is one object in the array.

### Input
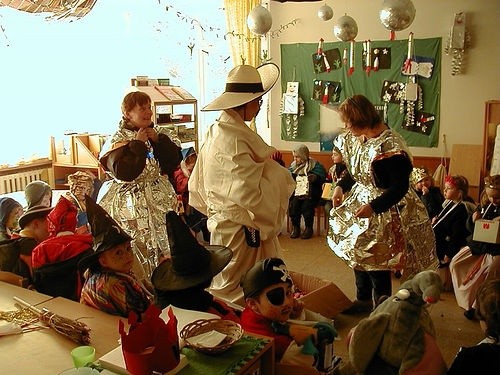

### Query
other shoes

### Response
[
  {"left": 301, "top": 227, "right": 314, "bottom": 239},
  {"left": 291, "top": 226, "right": 301, "bottom": 238},
  {"left": 341, "top": 301, "right": 374, "bottom": 315}
]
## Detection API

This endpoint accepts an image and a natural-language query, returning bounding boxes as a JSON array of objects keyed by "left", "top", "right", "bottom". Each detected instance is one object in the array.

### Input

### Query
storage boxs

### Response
[{"left": 231, "top": 271, "right": 354, "bottom": 318}]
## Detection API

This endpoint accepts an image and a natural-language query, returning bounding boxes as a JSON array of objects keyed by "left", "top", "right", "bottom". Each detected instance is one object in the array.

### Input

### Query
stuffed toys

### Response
[{"left": 336, "top": 270, "right": 447, "bottom": 375}]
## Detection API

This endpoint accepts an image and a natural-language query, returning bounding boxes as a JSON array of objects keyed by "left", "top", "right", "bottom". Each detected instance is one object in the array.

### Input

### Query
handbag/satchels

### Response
[{"left": 449, "top": 245, "right": 493, "bottom": 311}]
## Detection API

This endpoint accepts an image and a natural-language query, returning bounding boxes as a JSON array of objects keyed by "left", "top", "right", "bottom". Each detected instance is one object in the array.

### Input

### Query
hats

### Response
[
  {"left": 78, "top": 193, "right": 134, "bottom": 271},
  {"left": 201, "top": 61, "right": 280, "bottom": 112},
  {"left": 151, "top": 210, "right": 233, "bottom": 290},
  {"left": 239, "top": 256, "right": 294, "bottom": 298},
  {"left": 19, "top": 205, "right": 55, "bottom": 229}
]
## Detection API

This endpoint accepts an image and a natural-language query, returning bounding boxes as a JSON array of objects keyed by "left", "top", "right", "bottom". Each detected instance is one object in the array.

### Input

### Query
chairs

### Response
[{"left": 287, "top": 201, "right": 327, "bottom": 236}]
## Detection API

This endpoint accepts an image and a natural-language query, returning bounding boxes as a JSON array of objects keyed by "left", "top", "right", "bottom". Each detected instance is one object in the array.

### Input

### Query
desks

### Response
[
  {"left": 0, "top": 280, "right": 274, "bottom": 375},
  {"left": 0, "top": 189, "right": 70, "bottom": 212}
]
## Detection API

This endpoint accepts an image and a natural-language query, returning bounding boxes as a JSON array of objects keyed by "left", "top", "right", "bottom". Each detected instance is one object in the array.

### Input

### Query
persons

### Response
[
  {"left": 93, "top": 91, "right": 185, "bottom": 305},
  {"left": 429, "top": 175, "right": 471, "bottom": 268},
  {"left": 0, "top": 204, "right": 56, "bottom": 292},
  {"left": 237, "top": 257, "right": 339, "bottom": 375},
  {"left": 445, "top": 278, "right": 500, "bottom": 375},
  {"left": 173, "top": 145, "right": 212, "bottom": 245},
  {"left": 270, "top": 150, "right": 286, "bottom": 167},
  {"left": 30, "top": 195, "right": 95, "bottom": 305},
  {"left": 0, "top": 197, "right": 24, "bottom": 241},
  {"left": 449, "top": 174, "right": 500, "bottom": 321},
  {"left": 324, "top": 94, "right": 413, "bottom": 316},
  {"left": 412, "top": 166, "right": 445, "bottom": 221},
  {"left": 24, "top": 180, "right": 53, "bottom": 208},
  {"left": 46, "top": 170, "right": 97, "bottom": 235},
  {"left": 79, "top": 193, "right": 153, "bottom": 322},
  {"left": 322, "top": 146, "right": 348, "bottom": 234},
  {"left": 140, "top": 210, "right": 240, "bottom": 325},
  {"left": 188, "top": 61, "right": 297, "bottom": 310},
  {"left": 284, "top": 144, "right": 326, "bottom": 240}
]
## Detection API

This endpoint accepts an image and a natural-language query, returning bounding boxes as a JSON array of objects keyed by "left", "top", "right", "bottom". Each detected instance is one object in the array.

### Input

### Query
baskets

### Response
[{"left": 180, "top": 318, "right": 244, "bottom": 355}]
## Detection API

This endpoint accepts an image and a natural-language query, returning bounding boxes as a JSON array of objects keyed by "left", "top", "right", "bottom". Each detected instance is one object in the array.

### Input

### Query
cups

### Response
[{"left": 70, "top": 346, "right": 96, "bottom": 367}]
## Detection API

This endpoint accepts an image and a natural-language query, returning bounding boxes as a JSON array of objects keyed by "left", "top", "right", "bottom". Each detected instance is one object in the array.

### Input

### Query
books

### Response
[{"left": 136, "top": 83, "right": 197, "bottom": 100}]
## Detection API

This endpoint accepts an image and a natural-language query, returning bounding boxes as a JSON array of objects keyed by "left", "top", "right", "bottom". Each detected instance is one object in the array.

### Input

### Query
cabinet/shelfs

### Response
[{"left": 131, "top": 85, "right": 199, "bottom": 154}]
